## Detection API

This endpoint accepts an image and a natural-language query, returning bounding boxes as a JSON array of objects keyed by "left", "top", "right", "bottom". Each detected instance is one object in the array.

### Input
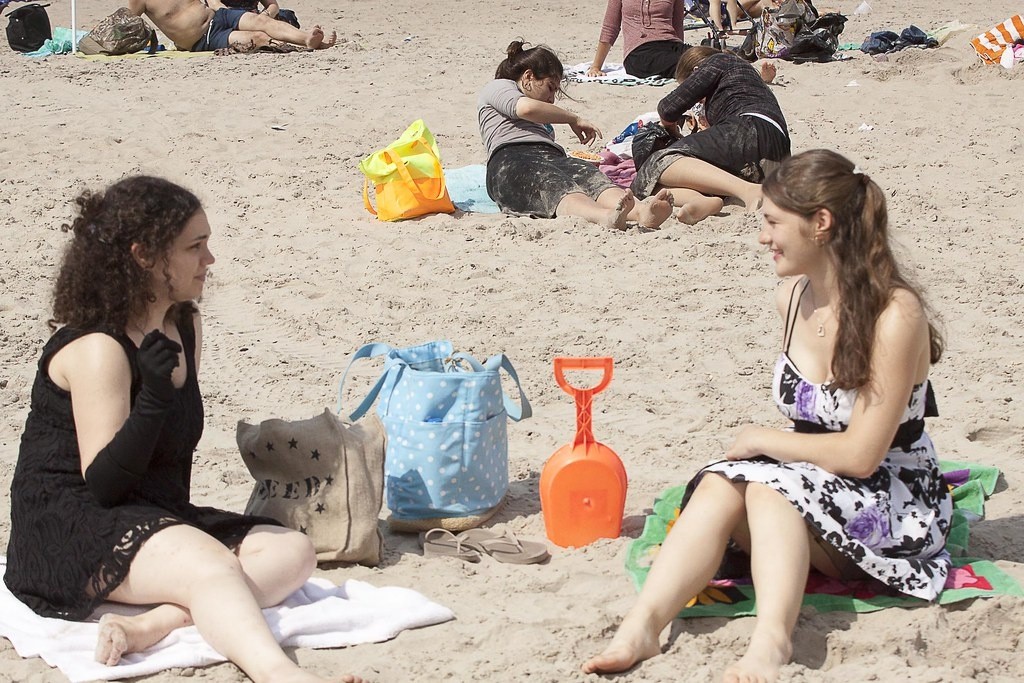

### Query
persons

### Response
[
  {"left": 579, "top": 146, "right": 949, "bottom": 683},
  {"left": 219, "top": 0, "right": 306, "bottom": 27},
  {"left": 2, "top": 171, "right": 365, "bottom": 683},
  {"left": 586, "top": 0, "right": 777, "bottom": 87},
  {"left": 125, "top": 0, "right": 337, "bottom": 53},
  {"left": 681, "top": 0, "right": 811, "bottom": 36},
  {"left": 473, "top": 35, "right": 675, "bottom": 230},
  {"left": 630, "top": 46, "right": 792, "bottom": 224}
]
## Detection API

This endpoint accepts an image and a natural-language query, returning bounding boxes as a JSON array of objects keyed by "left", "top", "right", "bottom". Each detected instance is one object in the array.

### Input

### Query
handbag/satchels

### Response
[
  {"left": 884, "top": 24, "right": 938, "bottom": 53},
  {"left": 78, "top": 6, "right": 158, "bottom": 56},
  {"left": 736, "top": 24, "right": 759, "bottom": 62},
  {"left": 274, "top": 8, "right": 300, "bottom": 30},
  {"left": 774, "top": 0, "right": 820, "bottom": 41},
  {"left": 4, "top": 2, "right": 54, "bottom": 52},
  {"left": 631, "top": 120, "right": 679, "bottom": 172},
  {"left": 359, "top": 118, "right": 455, "bottom": 222},
  {"left": 754, "top": 6, "right": 794, "bottom": 60},
  {"left": 334, "top": 339, "right": 533, "bottom": 533},
  {"left": 234, "top": 404, "right": 386, "bottom": 568},
  {"left": 789, "top": 11, "right": 848, "bottom": 63},
  {"left": 860, "top": 30, "right": 899, "bottom": 55}
]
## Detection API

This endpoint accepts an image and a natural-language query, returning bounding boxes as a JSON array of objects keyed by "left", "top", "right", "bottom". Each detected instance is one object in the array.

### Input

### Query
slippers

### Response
[
  {"left": 416, "top": 526, "right": 480, "bottom": 563},
  {"left": 254, "top": 45, "right": 302, "bottom": 53},
  {"left": 214, "top": 39, "right": 257, "bottom": 56},
  {"left": 455, "top": 528, "right": 550, "bottom": 566}
]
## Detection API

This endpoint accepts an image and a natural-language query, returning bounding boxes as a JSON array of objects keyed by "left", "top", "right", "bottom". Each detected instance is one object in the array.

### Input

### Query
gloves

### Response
[{"left": 84, "top": 326, "right": 184, "bottom": 508}]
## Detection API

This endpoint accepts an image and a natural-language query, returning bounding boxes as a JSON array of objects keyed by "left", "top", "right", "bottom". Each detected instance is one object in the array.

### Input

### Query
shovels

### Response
[{"left": 539, "top": 356, "right": 628, "bottom": 550}]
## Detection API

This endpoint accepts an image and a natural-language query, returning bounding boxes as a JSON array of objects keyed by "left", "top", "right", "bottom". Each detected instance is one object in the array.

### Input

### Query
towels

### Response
[
  {"left": 623, "top": 461, "right": 1023, "bottom": 619},
  {"left": 561, "top": 61, "right": 678, "bottom": 87},
  {"left": 595, "top": 149, "right": 638, "bottom": 187},
  {"left": 21, "top": 26, "right": 215, "bottom": 59},
  {"left": 443, "top": 163, "right": 501, "bottom": 214},
  {"left": 969, "top": 14, "right": 1024, "bottom": 64},
  {"left": 0, "top": 551, "right": 456, "bottom": 682}
]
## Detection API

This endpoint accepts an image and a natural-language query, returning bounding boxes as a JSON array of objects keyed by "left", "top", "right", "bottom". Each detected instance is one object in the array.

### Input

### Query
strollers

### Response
[{"left": 683, "top": 0, "right": 764, "bottom": 57}]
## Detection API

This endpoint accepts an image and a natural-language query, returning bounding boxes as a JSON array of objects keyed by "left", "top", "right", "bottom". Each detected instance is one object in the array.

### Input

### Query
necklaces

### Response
[
  {"left": 131, "top": 321, "right": 170, "bottom": 338},
  {"left": 807, "top": 277, "right": 846, "bottom": 338}
]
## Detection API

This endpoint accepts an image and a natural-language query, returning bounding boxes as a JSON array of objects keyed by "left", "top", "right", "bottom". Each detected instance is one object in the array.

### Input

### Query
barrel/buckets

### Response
[{"left": 568, "top": 151, "right": 606, "bottom": 172}]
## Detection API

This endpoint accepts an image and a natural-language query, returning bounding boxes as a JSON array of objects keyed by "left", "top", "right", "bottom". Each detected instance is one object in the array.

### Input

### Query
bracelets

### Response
[{"left": 263, "top": 9, "right": 269, "bottom": 16}]
